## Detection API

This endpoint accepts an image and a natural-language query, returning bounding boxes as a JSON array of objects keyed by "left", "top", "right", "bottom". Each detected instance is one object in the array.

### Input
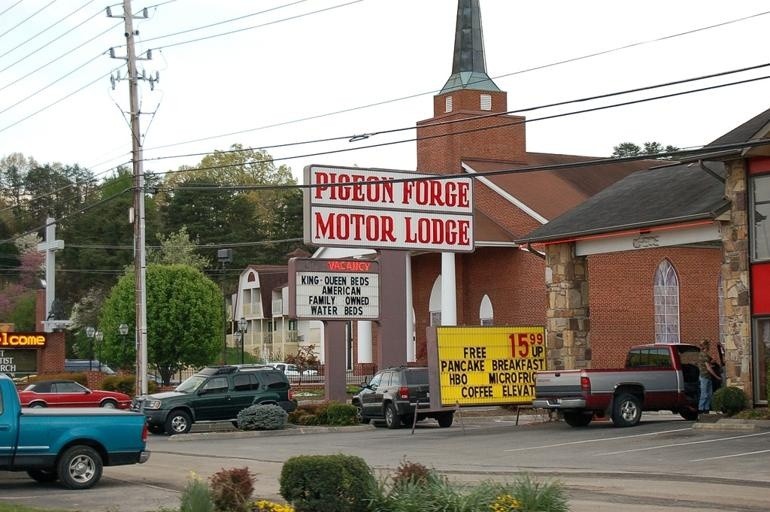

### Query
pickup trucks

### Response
[
  {"left": 0, "top": 372, "right": 151, "bottom": 490},
  {"left": 532, "top": 343, "right": 720, "bottom": 427}
]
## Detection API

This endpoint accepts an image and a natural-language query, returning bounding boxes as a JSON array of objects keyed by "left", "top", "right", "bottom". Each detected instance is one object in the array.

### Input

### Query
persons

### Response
[{"left": 697, "top": 339, "right": 722, "bottom": 414}]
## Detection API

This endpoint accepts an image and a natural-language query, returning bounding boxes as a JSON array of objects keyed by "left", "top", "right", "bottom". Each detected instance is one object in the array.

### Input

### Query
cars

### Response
[
  {"left": 18, "top": 379, "right": 132, "bottom": 409},
  {"left": 270, "top": 363, "right": 318, "bottom": 383}
]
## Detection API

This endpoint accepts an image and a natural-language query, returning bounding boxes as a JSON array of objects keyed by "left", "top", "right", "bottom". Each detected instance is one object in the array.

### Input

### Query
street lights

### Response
[
  {"left": 238, "top": 317, "right": 248, "bottom": 364},
  {"left": 86, "top": 323, "right": 128, "bottom": 372}
]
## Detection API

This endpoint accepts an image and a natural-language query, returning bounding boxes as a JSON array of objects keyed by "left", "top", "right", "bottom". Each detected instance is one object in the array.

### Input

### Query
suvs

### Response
[
  {"left": 130, "top": 364, "right": 297, "bottom": 435},
  {"left": 353, "top": 366, "right": 453, "bottom": 429}
]
